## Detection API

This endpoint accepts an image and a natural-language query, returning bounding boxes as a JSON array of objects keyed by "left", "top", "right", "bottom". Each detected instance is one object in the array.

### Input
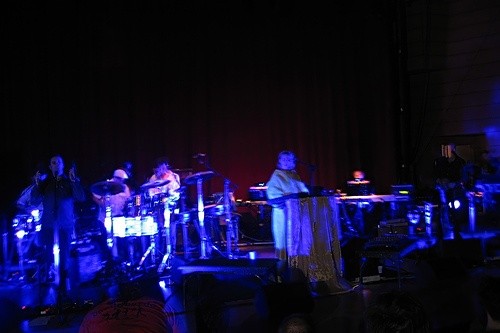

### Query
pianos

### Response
[{"left": 336, "top": 194, "right": 410, "bottom": 239}]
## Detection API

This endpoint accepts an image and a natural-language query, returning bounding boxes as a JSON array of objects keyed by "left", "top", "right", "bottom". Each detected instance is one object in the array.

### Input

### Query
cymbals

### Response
[
  {"left": 183, "top": 171, "right": 214, "bottom": 185},
  {"left": 141, "top": 179, "right": 170, "bottom": 188},
  {"left": 91, "top": 180, "right": 125, "bottom": 197}
]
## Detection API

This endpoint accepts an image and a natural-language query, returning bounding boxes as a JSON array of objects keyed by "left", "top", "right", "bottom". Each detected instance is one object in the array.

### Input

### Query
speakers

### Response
[
  {"left": 181, "top": 268, "right": 318, "bottom": 332},
  {"left": 358, "top": 281, "right": 486, "bottom": 333}
]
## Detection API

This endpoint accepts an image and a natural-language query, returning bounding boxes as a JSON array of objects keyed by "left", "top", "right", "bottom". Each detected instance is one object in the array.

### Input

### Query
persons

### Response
[
  {"left": 33, "top": 155, "right": 93, "bottom": 316},
  {"left": 94, "top": 169, "right": 132, "bottom": 260},
  {"left": 78, "top": 285, "right": 176, "bottom": 333},
  {"left": 267, "top": 151, "right": 311, "bottom": 260},
  {"left": 278, "top": 314, "right": 314, "bottom": 333},
  {"left": 359, "top": 288, "right": 433, "bottom": 333},
  {"left": 16, "top": 166, "right": 50, "bottom": 249},
  {"left": 146, "top": 158, "right": 180, "bottom": 256},
  {"left": 340, "top": 170, "right": 376, "bottom": 197},
  {"left": 477, "top": 278, "right": 500, "bottom": 332}
]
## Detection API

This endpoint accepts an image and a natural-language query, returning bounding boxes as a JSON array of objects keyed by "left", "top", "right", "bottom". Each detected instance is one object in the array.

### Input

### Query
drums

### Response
[
  {"left": 123, "top": 195, "right": 159, "bottom": 236},
  {"left": 213, "top": 192, "right": 236, "bottom": 225},
  {"left": 59, "top": 236, "right": 109, "bottom": 285}
]
